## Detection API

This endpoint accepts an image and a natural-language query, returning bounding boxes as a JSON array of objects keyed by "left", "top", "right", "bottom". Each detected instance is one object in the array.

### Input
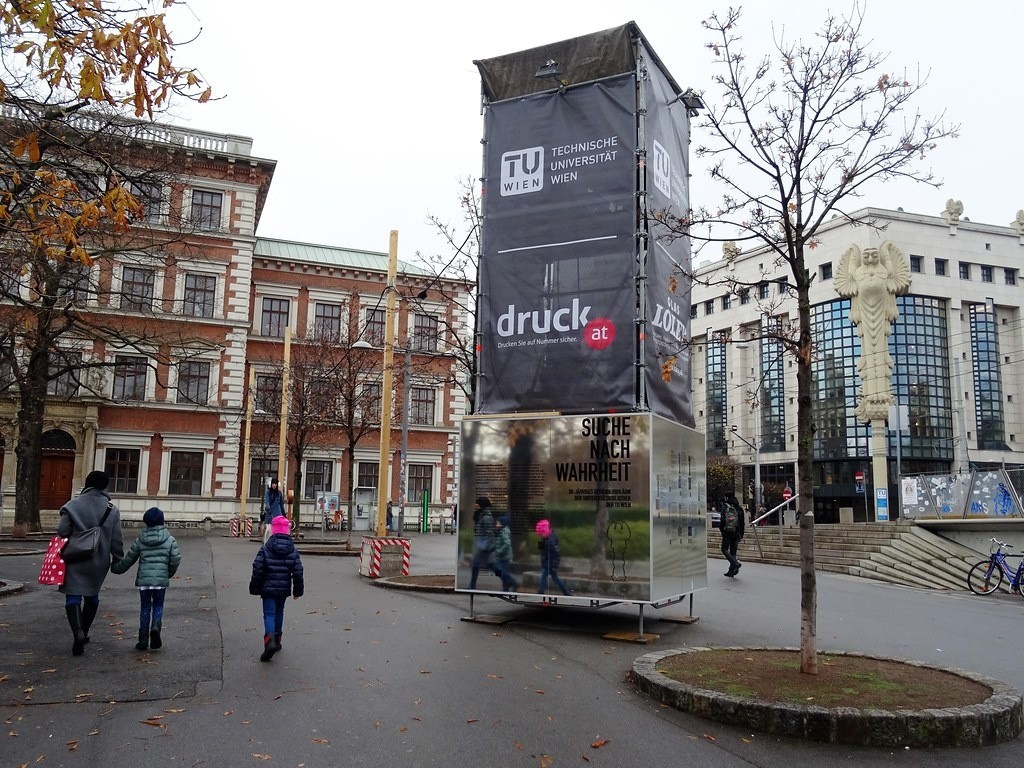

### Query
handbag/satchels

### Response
[
  {"left": 38, "top": 535, "right": 69, "bottom": 585},
  {"left": 61, "top": 527, "right": 100, "bottom": 562}
]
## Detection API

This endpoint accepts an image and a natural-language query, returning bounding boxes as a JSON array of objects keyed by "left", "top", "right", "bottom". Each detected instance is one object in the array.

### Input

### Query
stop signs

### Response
[
  {"left": 782, "top": 490, "right": 791, "bottom": 501},
  {"left": 856, "top": 471, "right": 863, "bottom": 481}
]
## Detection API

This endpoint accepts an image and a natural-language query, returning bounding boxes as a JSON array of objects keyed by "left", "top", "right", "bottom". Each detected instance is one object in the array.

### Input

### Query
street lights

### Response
[
  {"left": 724, "top": 425, "right": 761, "bottom": 525},
  {"left": 353, "top": 341, "right": 412, "bottom": 538}
]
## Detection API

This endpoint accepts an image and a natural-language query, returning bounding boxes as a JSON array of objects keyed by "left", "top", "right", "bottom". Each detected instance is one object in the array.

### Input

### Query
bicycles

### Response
[
  {"left": 967, "top": 537, "right": 1024, "bottom": 597},
  {"left": 338, "top": 512, "right": 348, "bottom": 531},
  {"left": 285, "top": 513, "right": 296, "bottom": 531},
  {"left": 324, "top": 511, "right": 334, "bottom": 530}
]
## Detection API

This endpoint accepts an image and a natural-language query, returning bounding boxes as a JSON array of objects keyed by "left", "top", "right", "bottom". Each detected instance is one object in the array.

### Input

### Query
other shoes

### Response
[
  {"left": 732, "top": 560, "right": 741, "bottom": 574},
  {"left": 724, "top": 571, "right": 734, "bottom": 577}
]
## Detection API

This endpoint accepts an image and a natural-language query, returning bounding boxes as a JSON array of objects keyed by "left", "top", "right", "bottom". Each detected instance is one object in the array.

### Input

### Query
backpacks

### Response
[{"left": 725, "top": 503, "right": 739, "bottom": 534}]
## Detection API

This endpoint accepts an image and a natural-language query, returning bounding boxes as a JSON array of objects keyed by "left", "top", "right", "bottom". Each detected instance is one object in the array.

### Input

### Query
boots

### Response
[
  {"left": 151, "top": 620, "right": 162, "bottom": 649},
  {"left": 65, "top": 604, "right": 85, "bottom": 655},
  {"left": 504, "top": 573, "right": 522, "bottom": 592},
  {"left": 259, "top": 631, "right": 282, "bottom": 660},
  {"left": 82, "top": 601, "right": 98, "bottom": 643},
  {"left": 136, "top": 628, "right": 148, "bottom": 650},
  {"left": 468, "top": 561, "right": 478, "bottom": 589}
]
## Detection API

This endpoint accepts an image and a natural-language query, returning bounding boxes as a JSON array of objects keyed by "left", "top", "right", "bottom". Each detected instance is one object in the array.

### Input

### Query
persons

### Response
[
  {"left": 536, "top": 520, "right": 573, "bottom": 597},
  {"left": 495, "top": 517, "right": 513, "bottom": 592},
  {"left": 248, "top": 515, "right": 304, "bottom": 663},
  {"left": 386, "top": 501, "right": 396, "bottom": 532},
  {"left": 464, "top": 496, "right": 520, "bottom": 592},
  {"left": 263, "top": 477, "right": 288, "bottom": 546},
  {"left": 55, "top": 471, "right": 125, "bottom": 656},
  {"left": 111, "top": 507, "right": 181, "bottom": 651},
  {"left": 719, "top": 492, "right": 745, "bottom": 578}
]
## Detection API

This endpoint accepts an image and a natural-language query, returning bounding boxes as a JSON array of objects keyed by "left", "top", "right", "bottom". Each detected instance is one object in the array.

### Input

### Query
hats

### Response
[
  {"left": 271, "top": 516, "right": 291, "bottom": 535},
  {"left": 143, "top": 507, "right": 164, "bottom": 526},
  {"left": 496, "top": 516, "right": 509, "bottom": 527},
  {"left": 476, "top": 497, "right": 491, "bottom": 508},
  {"left": 536, "top": 520, "right": 550, "bottom": 538},
  {"left": 85, "top": 471, "right": 109, "bottom": 490}
]
